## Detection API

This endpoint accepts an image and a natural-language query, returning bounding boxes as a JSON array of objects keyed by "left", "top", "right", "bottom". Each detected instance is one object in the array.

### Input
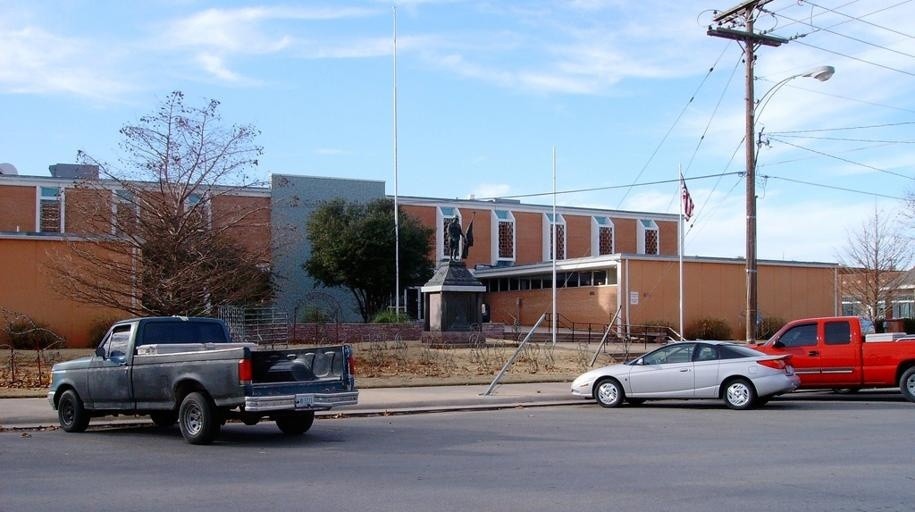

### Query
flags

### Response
[
  {"left": 681, "top": 175, "right": 695, "bottom": 221},
  {"left": 461, "top": 219, "right": 477, "bottom": 259}
]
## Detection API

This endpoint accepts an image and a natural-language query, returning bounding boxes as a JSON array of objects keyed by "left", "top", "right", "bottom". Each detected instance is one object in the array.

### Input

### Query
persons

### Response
[{"left": 447, "top": 214, "right": 463, "bottom": 259}]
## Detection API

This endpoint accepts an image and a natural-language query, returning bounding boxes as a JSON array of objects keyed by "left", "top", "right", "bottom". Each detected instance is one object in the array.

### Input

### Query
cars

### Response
[{"left": 570, "top": 339, "right": 802, "bottom": 408}]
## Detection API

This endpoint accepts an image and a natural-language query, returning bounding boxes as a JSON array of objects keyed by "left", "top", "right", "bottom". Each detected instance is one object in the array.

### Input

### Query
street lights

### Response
[{"left": 746, "top": 63, "right": 836, "bottom": 344}]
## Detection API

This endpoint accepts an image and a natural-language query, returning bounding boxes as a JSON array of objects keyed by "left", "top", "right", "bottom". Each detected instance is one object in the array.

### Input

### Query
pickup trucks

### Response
[
  {"left": 742, "top": 316, "right": 914, "bottom": 401},
  {"left": 47, "top": 316, "right": 360, "bottom": 445}
]
